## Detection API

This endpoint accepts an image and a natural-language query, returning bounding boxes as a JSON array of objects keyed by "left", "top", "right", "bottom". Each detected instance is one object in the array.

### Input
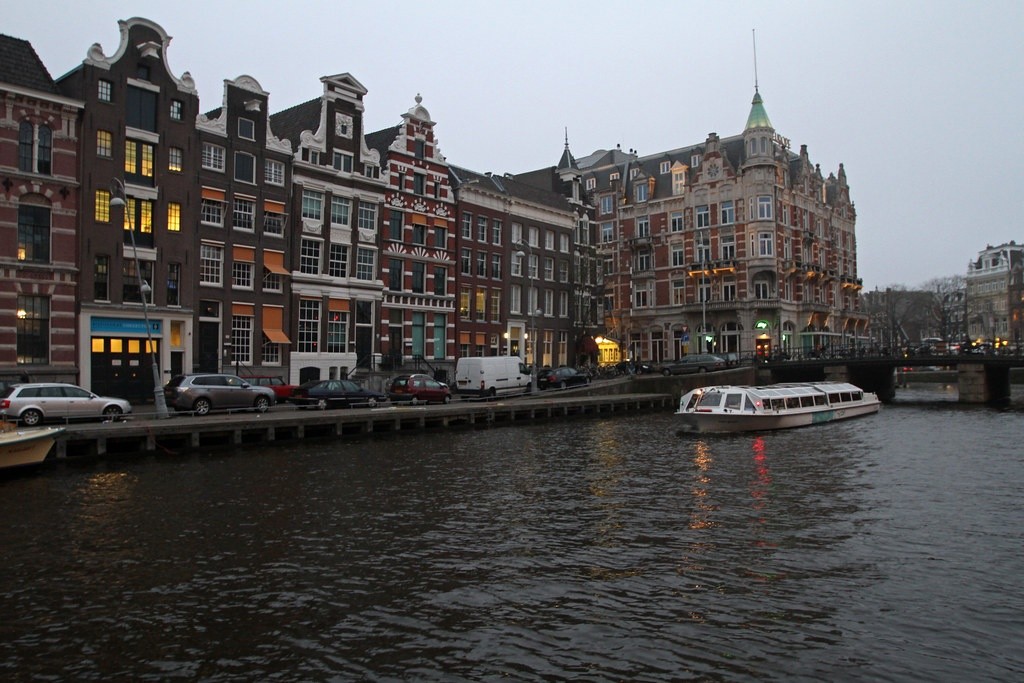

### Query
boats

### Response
[{"left": 674, "top": 380, "right": 882, "bottom": 435}]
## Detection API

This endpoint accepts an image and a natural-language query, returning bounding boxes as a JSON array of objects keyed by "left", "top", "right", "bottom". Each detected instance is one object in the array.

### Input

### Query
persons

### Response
[
  {"left": 635, "top": 356, "right": 643, "bottom": 375},
  {"left": 964, "top": 335, "right": 972, "bottom": 353},
  {"left": 904, "top": 343, "right": 911, "bottom": 358},
  {"left": 816, "top": 343, "right": 826, "bottom": 358},
  {"left": 841, "top": 345, "right": 893, "bottom": 358}
]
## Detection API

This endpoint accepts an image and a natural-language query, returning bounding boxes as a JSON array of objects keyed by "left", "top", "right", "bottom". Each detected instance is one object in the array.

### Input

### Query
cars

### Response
[
  {"left": 289, "top": 379, "right": 388, "bottom": 412},
  {"left": 655, "top": 354, "right": 726, "bottom": 377},
  {"left": 1, "top": 383, "right": 132, "bottom": 426},
  {"left": 616, "top": 362, "right": 655, "bottom": 374},
  {"left": 536, "top": 367, "right": 592, "bottom": 391},
  {"left": 921, "top": 337, "right": 996, "bottom": 354}
]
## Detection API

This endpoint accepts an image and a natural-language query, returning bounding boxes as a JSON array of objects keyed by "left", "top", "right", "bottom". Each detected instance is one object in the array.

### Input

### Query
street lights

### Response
[
  {"left": 697, "top": 229, "right": 711, "bottom": 355},
  {"left": 514, "top": 238, "right": 540, "bottom": 396},
  {"left": 107, "top": 177, "right": 170, "bottom": 419}
]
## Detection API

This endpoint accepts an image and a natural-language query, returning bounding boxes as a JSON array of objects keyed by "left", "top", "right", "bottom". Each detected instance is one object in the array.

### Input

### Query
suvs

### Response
[
  {"left": 237, "top": 375, "right": 300, "bottom": 404},
  {"left": 160, "top": 373, "right": 278, "bottom": 417},
  {"left": 388, "top": 373, "right": 452, "bottom": 406}
]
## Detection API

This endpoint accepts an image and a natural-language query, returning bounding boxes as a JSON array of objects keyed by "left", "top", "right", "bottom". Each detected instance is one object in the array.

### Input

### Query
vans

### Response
[{"left": 454, "top": 356, "right": 532, "bottom": 402}]
